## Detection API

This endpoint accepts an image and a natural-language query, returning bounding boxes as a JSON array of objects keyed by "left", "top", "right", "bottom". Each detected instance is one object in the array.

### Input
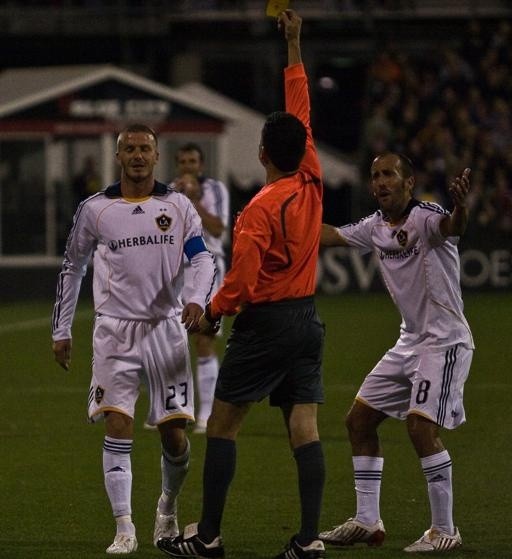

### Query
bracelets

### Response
[{"left": 205, "top": 302, "right": 221, "bottom": 323}]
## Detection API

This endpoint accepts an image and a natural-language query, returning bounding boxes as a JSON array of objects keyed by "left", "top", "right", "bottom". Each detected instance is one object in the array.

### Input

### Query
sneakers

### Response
[
  {"left": 157, "top": 533, "right": 225, "bottom": 559},
  {"left": 152, "top": 494, "right": 179, "bottom": 547},
  {"left": 318, "top": 516, "right": 386, "bottom": 547},
  {"left": 105, "top": 533, "right": 138, "bottom": 555},
  {"left": 272, "top": 533, "right": 328, "bottom": 559},
  {"left": 402, "top": 526, "right": 464, "bottom": 553},
  {"left": 192, "top": 415, "right": 209, "bottom": 435}
]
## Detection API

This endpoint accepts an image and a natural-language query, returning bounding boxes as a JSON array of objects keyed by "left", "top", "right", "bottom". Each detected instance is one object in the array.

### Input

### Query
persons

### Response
[
  {"left": 164, "top": 140, "right": 230, "bottom": 435},
  {"left": 352, "top": 21, "right": 511, "bottom": 230},
  {"left": 153, "top": 5, "right": 329, "bottom": 559},
  {"left": 315, "top": 148, "right": 476, "bottom": 552},
  {"left": 52, "top": 122, "right": 218, "bottom": 554}
]
